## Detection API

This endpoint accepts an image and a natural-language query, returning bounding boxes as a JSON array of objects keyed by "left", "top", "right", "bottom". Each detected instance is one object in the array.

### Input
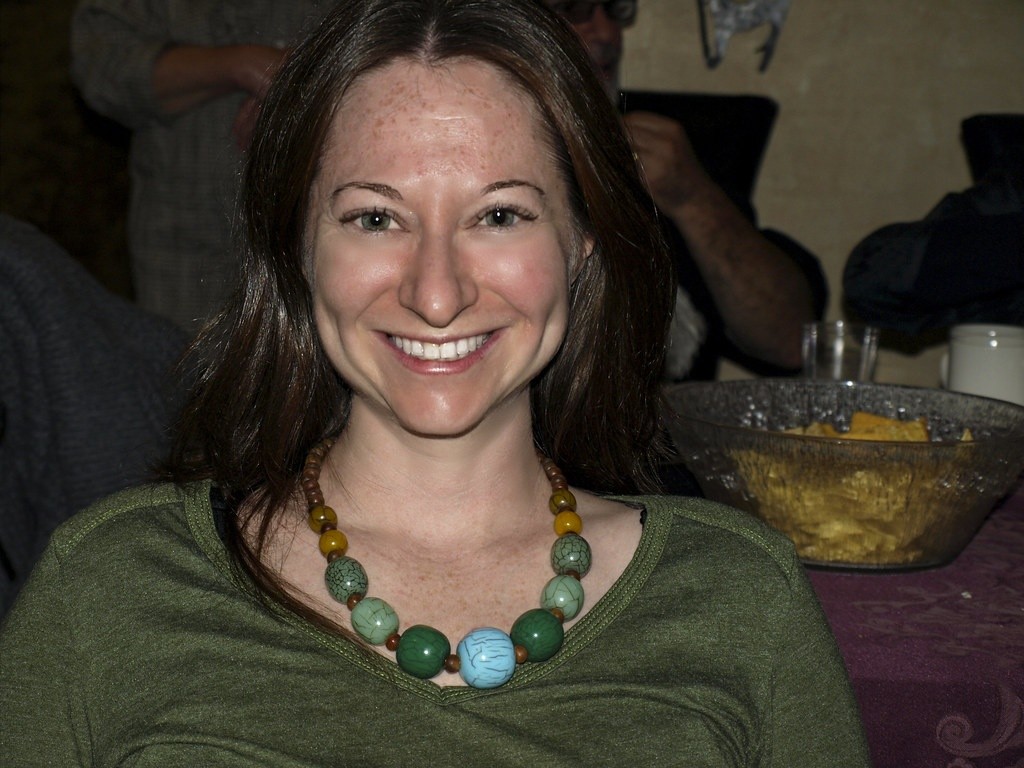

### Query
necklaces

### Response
[{"left": 299, "top": 425, "right": 593, "bottom": 687}]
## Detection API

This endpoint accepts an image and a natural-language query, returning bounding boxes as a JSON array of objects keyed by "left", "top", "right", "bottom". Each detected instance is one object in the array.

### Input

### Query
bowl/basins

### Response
[{"left": 666, "top": 380, "right": 1024, "bottom": 571}]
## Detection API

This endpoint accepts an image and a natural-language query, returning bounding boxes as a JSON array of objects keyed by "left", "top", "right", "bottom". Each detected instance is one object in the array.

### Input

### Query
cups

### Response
[
  {"left": 952, "top": 324, "right": 1024, "bottom": 340},
  {"left": 802, "top": 322, "right": 879, "bottom": 383},
  {"left": 951, "top": 337, "right": 1024, "bottom": 408}
]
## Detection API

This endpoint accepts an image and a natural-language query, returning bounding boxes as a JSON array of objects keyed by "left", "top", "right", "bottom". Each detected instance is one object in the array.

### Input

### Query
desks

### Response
[{"left": 656, "top": 467, "right": 1024, "bottom": 768}]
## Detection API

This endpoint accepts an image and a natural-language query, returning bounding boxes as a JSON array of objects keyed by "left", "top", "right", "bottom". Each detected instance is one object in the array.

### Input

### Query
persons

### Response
[
  {"left": 524, "top": 0, "right": 836, "bottom": 376},
  {"left": 1, "top": 0, "right": 868, "bottom": 768},
  {"left": 66, "top": 0, "right": 350, "bottom": 369}
]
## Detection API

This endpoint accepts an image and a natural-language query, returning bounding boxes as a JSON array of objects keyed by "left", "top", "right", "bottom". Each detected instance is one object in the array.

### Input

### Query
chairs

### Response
[{"left": 619, "top": 87, "right": 783, "bottom": 201}]
